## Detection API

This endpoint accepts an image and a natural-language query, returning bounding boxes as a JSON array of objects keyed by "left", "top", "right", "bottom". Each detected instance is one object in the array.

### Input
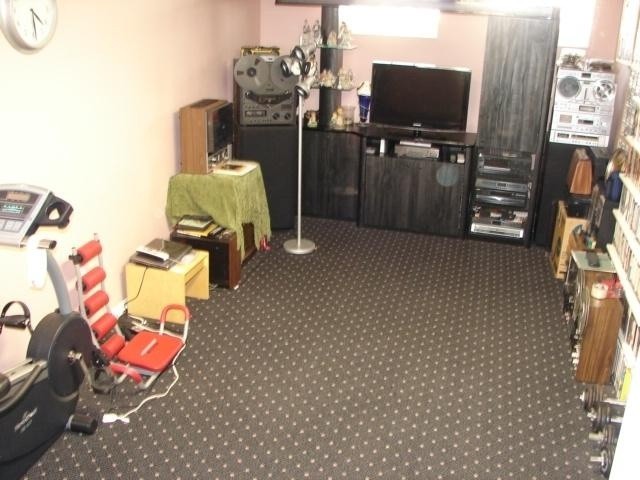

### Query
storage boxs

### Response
[{"left": 544, "top": 199, "right": 587, "bottom": 277}]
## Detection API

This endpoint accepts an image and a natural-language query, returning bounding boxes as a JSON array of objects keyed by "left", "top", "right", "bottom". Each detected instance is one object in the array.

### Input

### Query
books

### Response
[
  {"left": 609, "top": 70, "right": 640, "bottom": 401},
  {"left": 176, "top": 217, "right": 218, "bottom": 238}
]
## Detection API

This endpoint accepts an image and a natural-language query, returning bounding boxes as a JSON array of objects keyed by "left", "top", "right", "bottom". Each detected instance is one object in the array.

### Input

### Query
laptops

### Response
[{"left": 129, "top": 237, "right": 193, "bottom": 271}]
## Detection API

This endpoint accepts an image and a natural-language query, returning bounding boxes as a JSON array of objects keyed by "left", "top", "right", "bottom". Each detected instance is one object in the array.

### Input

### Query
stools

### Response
[{"left": 125, "top": 249, "right": 214, "bottom": 324}]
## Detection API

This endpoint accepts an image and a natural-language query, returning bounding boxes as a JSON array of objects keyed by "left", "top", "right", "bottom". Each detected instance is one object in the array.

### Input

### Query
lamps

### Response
[{"left": 281, "top": 36, "right": 320, "bottom": 256}]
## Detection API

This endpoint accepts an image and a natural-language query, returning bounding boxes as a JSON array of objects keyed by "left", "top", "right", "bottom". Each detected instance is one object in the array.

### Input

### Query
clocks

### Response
[{"left": 2, "top": 0, "right": 59, "bottom": 52}]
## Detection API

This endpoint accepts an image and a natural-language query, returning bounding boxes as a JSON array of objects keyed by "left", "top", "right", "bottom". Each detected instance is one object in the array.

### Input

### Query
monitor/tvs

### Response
[{"left": 369, "top": 61, "right": 471, "bottom": 147}]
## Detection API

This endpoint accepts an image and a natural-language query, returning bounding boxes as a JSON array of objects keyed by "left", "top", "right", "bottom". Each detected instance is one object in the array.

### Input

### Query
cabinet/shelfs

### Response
[
  {"left": 566, "top": 61, "right": 639, "bottom": 480},
  {"left": 171, "top": 160, "right": 266, "bottom": 262},
  {"left": 273, "top": 0, "right": 363, "bottom": 219},
  {"left": 468, "top": 14, "right": 558, "bottom": 248},
  {"left": 354, "top": 121, "right": 478, "bottom": 242}
]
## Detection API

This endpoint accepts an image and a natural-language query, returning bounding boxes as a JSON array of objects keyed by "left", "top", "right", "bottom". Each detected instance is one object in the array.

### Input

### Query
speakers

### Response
[
  {"left": 548, "top": 67, "right": 617, "bottom": 148},
  {"left": 235, "top": 53, "right": 299, "bottom": 126},
  {"left": 179, "top": 98, "right": 235, "bottom": 176}
]
